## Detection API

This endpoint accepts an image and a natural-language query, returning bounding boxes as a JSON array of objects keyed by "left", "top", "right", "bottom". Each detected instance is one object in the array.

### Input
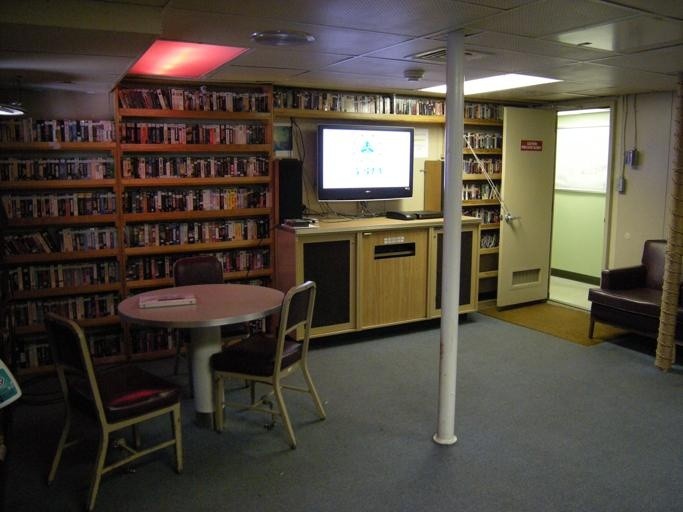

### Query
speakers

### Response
[{"left": 279, "top": 157, "right": 303, "bottom": 224}]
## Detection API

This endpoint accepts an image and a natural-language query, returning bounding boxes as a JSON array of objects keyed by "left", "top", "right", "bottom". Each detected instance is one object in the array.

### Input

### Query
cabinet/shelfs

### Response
[
  {"left": 116, "top": 84, "right": 278, "bottom": 361},
  {"left": 278, "top": 222, "right": 490, "bottom": 341},
  {"left": 0, "top": 108, "right": 120, "bottom": 378},
  {"left": 453, "top": 99, "right": 499, "bottom": 307},
  {"left": 273, "top": 85, "right": 449, "bottom": 120}
]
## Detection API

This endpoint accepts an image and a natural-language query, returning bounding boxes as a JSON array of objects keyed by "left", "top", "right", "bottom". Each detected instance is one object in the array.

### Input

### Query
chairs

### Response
[
  {"left": 44, "top": 311, "right": 188, "bottom": 510},
  {"left": 169, "top": 256, "right": 252, "bottom": 411},
  {"left": 206, "top": 279, "right": 325, "bottom": 451},
  {"left": 588, "top": 240, "right": 682, "bottom": 361}
]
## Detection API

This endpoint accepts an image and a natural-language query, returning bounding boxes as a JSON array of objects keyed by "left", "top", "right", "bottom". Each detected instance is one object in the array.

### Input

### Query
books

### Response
[
  {"left": 461, "top": 99, "right": 501, "bottom": 248},
  {"left": 119, "top": 86, "right": 271, "bottom": 291},
  {"left": 130, "top": 317, "right": 267, "bottom": 352},
  {"left": 272, "top": 85, "right": 447, "bottom": 117},
  {"left": 1, "top": 113, "right": 122, "bottom": 369}
]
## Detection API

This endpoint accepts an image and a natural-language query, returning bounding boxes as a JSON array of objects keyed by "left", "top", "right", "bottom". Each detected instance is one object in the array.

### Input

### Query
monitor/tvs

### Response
[{"left": 315, "top": 124, "right": 415, "bottom": 202}]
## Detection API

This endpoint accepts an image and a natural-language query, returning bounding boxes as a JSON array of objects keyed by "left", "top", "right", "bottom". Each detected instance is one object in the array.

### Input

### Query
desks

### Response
[{"left": 115, "top": 281, "right": 290, "bottom": 404}]
